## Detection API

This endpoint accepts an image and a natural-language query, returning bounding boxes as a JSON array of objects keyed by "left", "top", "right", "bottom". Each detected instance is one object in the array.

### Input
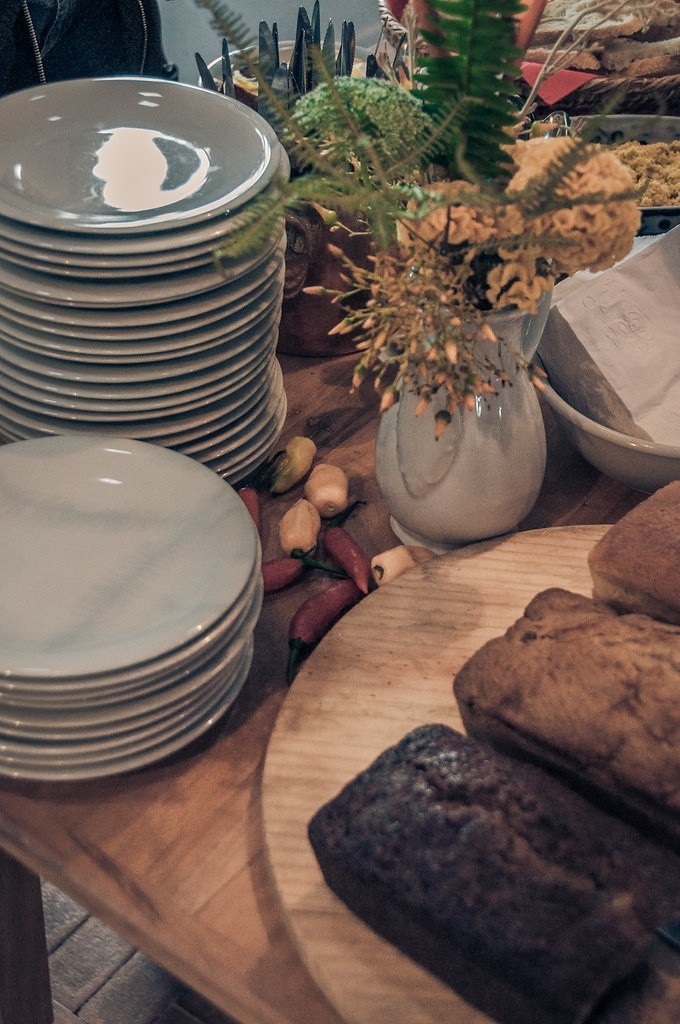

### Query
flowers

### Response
[{"left": 300, "top": 136, "right": 645, "bottom": 442}]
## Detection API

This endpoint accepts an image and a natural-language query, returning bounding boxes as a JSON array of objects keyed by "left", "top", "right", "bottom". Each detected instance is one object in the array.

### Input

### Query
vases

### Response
[
  {"left": 276, "top": 175, "right": 400, "bottom": 359},
  {"left": 373, "top": 255, "right": 554, "bottom": 556},
  {"left": 530, "top": 113, "right": 680, "bottom": 236}
]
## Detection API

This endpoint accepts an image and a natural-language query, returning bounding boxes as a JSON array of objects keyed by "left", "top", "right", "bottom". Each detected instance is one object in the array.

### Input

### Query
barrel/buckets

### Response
[{"left": 200, "top": 35, "right": 399, "bottom": 356}]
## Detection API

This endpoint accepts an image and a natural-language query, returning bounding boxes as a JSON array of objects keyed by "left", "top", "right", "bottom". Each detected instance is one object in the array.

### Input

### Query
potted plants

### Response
[{"left": 195, "top": 0, "right": 412, "bottom": 119}]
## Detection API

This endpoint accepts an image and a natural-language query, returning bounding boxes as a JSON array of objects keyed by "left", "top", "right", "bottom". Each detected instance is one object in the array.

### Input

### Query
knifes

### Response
[{"left": 195, "top": 1, "right": 407, "bottom": 133}]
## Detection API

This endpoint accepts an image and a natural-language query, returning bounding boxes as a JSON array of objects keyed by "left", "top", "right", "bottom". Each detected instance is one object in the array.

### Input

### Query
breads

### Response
[
  {"left": 523, "top": 0, "right": 680, "bottom": 79},
  {"left": 306, "top": 478, "right": 680, "bottom": 1024}
]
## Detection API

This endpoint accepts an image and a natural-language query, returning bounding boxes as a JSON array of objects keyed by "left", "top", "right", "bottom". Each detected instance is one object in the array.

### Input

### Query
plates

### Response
[{"left": 0, "top": 74, "right": 290, "bottom": 782}]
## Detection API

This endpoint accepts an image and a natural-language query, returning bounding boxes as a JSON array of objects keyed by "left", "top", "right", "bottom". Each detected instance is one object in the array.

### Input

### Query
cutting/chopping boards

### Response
[{"left": 259, "top": 525, "right": 627, "bottom": 1023}]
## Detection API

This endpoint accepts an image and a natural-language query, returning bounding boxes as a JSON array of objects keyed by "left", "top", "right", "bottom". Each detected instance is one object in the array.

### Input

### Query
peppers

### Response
[{"left": 238, "top": 431, "right": 444, "bottom": 683}]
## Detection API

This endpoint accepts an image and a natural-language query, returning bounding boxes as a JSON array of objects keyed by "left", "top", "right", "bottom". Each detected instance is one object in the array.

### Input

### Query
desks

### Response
[{"left": 0, "top": 311, "right": 654, "bottom": 1024}]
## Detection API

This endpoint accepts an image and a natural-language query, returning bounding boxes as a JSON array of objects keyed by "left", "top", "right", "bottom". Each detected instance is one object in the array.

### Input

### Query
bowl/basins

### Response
[
  {"left": 529, "top": 351, "right": 680, "bottom": 495},
  {"left": 566, "top": 116, "right": 680, "bottom": 234}
]
